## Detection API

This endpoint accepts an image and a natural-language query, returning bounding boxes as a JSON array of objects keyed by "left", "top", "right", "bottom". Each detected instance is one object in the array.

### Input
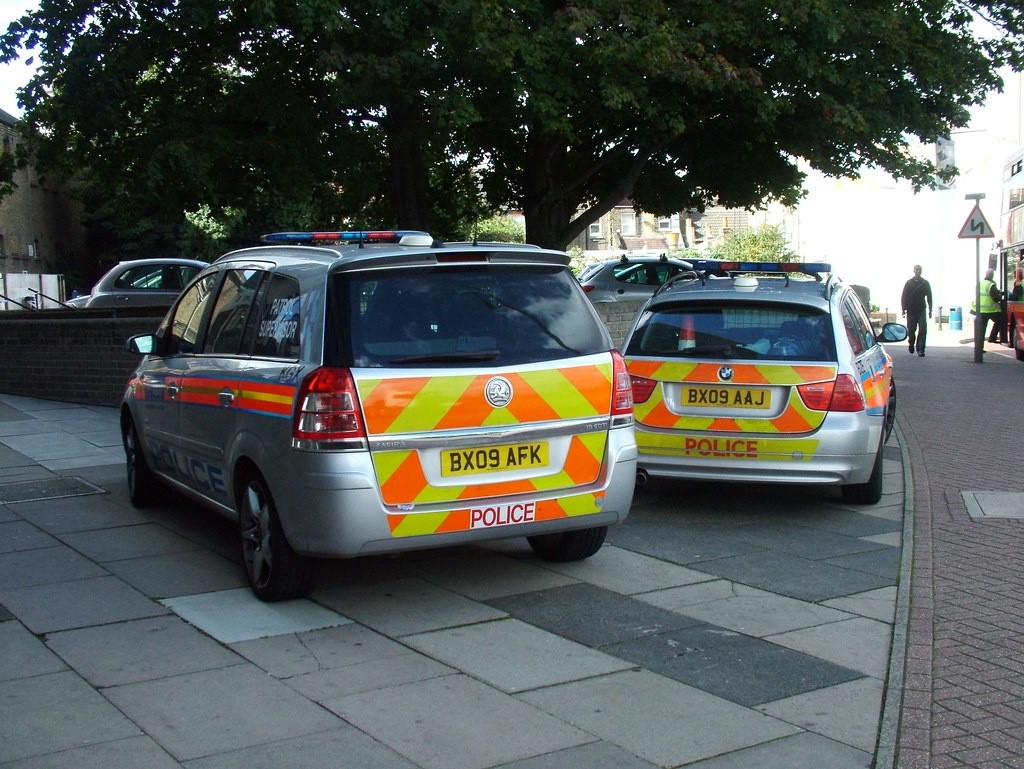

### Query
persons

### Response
[
  {"left": 902, "top": 265, "right": 932, "bottom": 356},
  {"left": 970, "top": 268, "right": 1003, "bottom": 353},
  {"left": 1007, "top": 268, "right": 1024, "bottom": 302}
]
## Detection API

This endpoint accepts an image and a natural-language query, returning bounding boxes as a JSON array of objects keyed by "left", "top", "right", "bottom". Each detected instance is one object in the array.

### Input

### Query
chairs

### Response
[
  {"left": 780, "top": 320, "right": 830, "bottom": 360},
  {"left": 693, "top": 310, "right": 724, "bottom": 331},
  {"left": 160, "top": 266, "right": 179, "bottom": 288}
]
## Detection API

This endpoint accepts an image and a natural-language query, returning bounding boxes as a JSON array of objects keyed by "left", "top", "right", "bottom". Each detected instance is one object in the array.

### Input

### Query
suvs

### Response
[
  {"left": 619, "top": 269, "right": 910, "bottom": 506},
  {"left": 118, "top": 227, "right": 636, "bottom": 603}
]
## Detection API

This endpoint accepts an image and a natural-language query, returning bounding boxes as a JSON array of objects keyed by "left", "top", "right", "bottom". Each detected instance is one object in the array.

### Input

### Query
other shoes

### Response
[
  {"left": 988, "top": 337, "right": 1000, "bottom": 343},
  {"left": 982, "top": 350, "right": 986, "bottom": 353}
]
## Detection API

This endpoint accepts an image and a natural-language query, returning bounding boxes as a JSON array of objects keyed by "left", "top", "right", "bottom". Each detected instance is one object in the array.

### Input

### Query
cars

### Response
[
  {"left": 578, "top": 258, "right": 721, "bottom": 304},
  {"left": 59, "top": 258, "right": 212, "bottom": 306}
]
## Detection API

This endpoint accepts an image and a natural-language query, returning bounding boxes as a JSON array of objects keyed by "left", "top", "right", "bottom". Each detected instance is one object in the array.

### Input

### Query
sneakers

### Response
[
  {"left": 909, "top": 345, "right": 914, "bottom": 353},
  {"left": 917, "top": 350, "right": 925, "bottom": 357}
]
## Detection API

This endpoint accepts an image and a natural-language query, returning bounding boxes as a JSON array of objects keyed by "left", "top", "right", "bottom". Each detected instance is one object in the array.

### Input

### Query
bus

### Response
[{"left": 996, "top": 148, "right": 1024, "bottom": 365}]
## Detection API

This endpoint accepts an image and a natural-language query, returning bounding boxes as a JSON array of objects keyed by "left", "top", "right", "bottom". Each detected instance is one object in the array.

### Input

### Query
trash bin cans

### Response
[{"left": 949, "top": 306, "right": 962, "bottom": 330}]
